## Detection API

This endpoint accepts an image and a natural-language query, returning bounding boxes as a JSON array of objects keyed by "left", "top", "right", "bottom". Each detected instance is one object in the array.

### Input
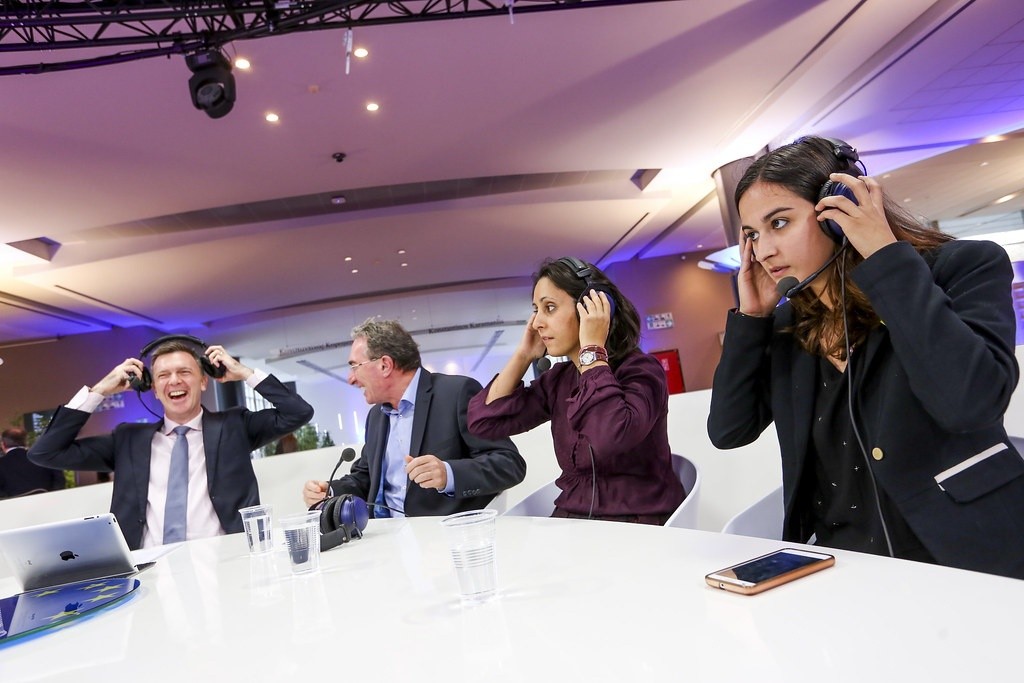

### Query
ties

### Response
[{"left": 162, "top": 426, "right": 191, "bottom": 544}]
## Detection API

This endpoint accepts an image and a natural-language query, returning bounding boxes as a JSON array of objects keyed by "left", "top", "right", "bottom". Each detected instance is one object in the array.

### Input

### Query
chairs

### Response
[
  {"left": 313, "top": 488, "right": 507, "bottom": 518},
  {"left": 501, "top": 453, "right": 700, "bottom": 531},
  {"left": 720, "top": 435, "right": 1024, "bottom": 545}
]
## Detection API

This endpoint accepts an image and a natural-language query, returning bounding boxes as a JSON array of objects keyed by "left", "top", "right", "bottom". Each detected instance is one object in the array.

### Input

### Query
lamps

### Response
[{"left": 186, "top": 49, "right": 236, "bottom": 119}]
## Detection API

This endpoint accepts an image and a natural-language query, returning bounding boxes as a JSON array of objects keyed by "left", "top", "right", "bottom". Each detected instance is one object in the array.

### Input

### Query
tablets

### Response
[
  {"left": 8, "top": 578, "right": 135, "bottom": 637},
  {"left": 0, "top": 512, "right": 140, "bottom": 592}
]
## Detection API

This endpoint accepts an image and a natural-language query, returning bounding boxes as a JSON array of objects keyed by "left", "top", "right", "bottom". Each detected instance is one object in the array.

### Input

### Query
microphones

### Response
[
  {"left": 777, "top": 240, "right": 851, "bottom": 298},
  {"left": 325, "top": 448, "right": 356, "bottom": 501},
  {"left": 130, "top": 374, "right": 140, "bottom": 389},
  {"left": 536, "top": 348, "right": 551, "bottom": 372}
]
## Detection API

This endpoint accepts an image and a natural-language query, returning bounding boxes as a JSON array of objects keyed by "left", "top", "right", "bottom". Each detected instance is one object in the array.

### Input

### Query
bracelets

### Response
[{"left": 579, "top": 345, "right": 607, "bottom": 355}]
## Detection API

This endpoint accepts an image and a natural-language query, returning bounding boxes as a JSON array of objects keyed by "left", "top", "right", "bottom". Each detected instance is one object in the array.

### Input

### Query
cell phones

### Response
[{"left": 705, "top": 547, "right": 835, "bottom": 596}]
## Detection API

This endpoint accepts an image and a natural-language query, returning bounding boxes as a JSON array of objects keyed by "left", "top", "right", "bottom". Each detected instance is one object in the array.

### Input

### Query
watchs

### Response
[{"left": 578, "top": 351, "right": 609, "bottom": 366}]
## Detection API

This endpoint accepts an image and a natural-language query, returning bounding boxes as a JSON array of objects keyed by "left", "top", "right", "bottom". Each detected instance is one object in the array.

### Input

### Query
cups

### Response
[
  {"left": 238, "top": 504, "right": 275, "bottom": 553},
  {"left": 444, "top": 508, "right": 499, "bottom": 604},
  {"left": 281, "top": 510, "right": 322, "bottom": 574}
]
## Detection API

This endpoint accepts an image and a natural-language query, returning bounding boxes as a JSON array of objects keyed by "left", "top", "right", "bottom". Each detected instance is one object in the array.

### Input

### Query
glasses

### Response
[{"left": 348, "top": 353, "right": 396, "bottom": 373}]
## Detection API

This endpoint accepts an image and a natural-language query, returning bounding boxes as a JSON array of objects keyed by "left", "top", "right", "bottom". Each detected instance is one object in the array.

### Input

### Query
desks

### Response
[{"left": 0, "top": 518, "right": 1024, "bottom": 683}]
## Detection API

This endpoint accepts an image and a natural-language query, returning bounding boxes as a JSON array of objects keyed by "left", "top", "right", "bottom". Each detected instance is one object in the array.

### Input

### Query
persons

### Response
[
  {"left": 302, "top": 318, "right": 527, "bottom": 519},
  {"left": 467, "top": 257, "right": 688, "bottom": 527},
  {"left": 0, "top": 427, "right": 65, "bottom": 500},
  {"left": 26, "top": 341, "right": 314, "bottom": 551},
  {"left": 707, "top": 136, "right": 1024, "bottom": 581}
]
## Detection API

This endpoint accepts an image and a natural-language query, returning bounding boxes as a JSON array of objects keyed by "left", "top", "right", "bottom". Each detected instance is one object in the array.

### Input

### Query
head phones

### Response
[
  {"left": 306, "top": 495, "right": 369, "bottom": 552},
  {"left": 797, "top": 135, "right": 871, "bottom": 246},
  {"left": 129, "top": 333, "right": 226, "bottom": 393},
  {"left": 555, "top": 255, "right": 616, "bottom": 326}
]
